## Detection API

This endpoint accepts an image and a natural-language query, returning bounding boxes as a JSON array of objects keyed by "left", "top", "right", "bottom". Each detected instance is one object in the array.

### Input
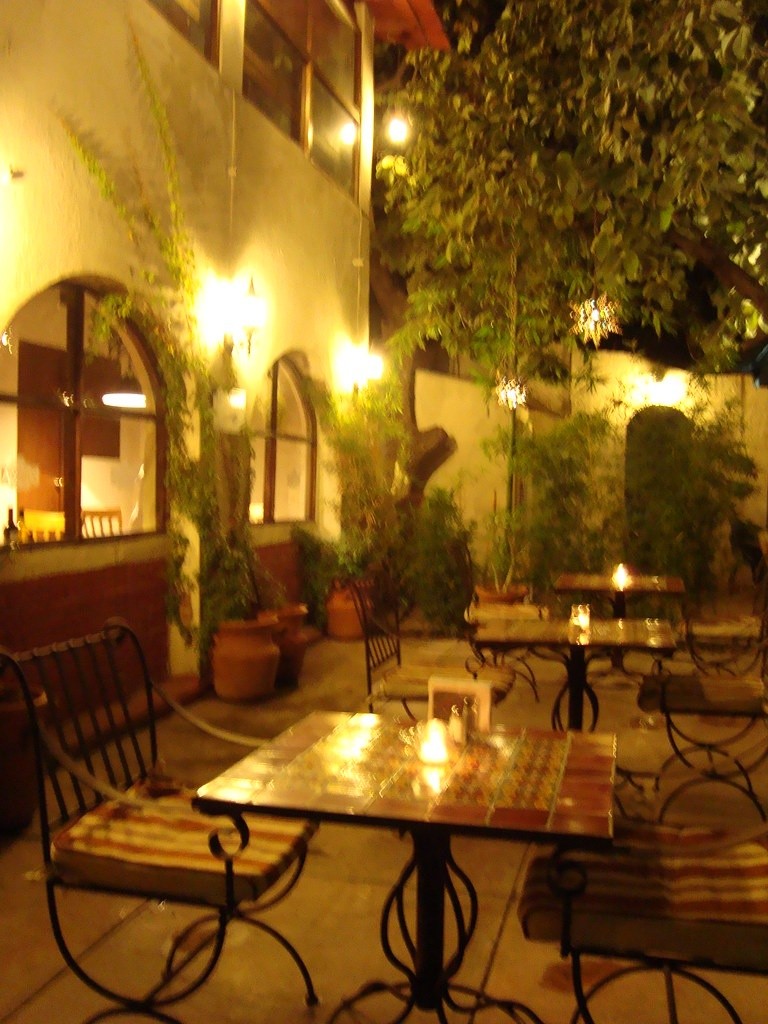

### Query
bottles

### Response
[
  {"left": 4, "top": 507, "right": 29, "bottom": 546},
  {"left": 450, "top": 697, "right": 479, "bottom": 744}
]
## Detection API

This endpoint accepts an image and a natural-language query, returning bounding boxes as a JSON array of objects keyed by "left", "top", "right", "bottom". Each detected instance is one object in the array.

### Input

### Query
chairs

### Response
[
  {"left": 354, "top": 582, "right": 513, "bottom": 717},
  {"left": 14, "top": 505, "right": 130, "bottom": 544},
  {"left": 0, "top": 619, "right": 323, "bottom": 1009},
  {"left": 463, "top": 516, "right": 768, "bottom": 1024}
]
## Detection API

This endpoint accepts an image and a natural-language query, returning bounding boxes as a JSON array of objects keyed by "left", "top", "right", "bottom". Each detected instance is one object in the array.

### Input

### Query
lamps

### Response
[{"left": 100, "top": 356, "right": 151, "bottom": 409}]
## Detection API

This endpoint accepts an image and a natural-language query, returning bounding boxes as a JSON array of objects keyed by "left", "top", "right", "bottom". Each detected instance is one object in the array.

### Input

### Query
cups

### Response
[{"left": 569, "top": 600, "right": 592, "bottom": 645}]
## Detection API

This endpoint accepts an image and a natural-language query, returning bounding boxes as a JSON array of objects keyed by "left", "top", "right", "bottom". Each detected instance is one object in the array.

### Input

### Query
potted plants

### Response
[
  {"left": 293, "top": 529, "right": 371, "bottom": 639},
  {"left": 208, "top": 549, "right": 284, "bottom": 699},
  {"left": 472, "top": 512, "right": 529, "bottom": 605},
  {"left": 243, "top": 539, "right": 309, "bottom": 684}
]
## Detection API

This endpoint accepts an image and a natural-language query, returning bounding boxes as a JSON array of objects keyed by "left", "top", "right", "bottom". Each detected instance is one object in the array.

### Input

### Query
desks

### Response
[
  {"left": 190, "top": 708, "right": 617, "bottom": 1023},
  {"left": 470, "top": 617, "right": 676, "bottom": 820},
  {"left": 551, "top": 571, "right": 686, "bottom": 685}
]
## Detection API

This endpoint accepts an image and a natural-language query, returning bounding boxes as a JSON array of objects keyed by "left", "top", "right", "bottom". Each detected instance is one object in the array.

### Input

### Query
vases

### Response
[{"left": 1, "top": 668, "right": 51, "bottom": 849}]
